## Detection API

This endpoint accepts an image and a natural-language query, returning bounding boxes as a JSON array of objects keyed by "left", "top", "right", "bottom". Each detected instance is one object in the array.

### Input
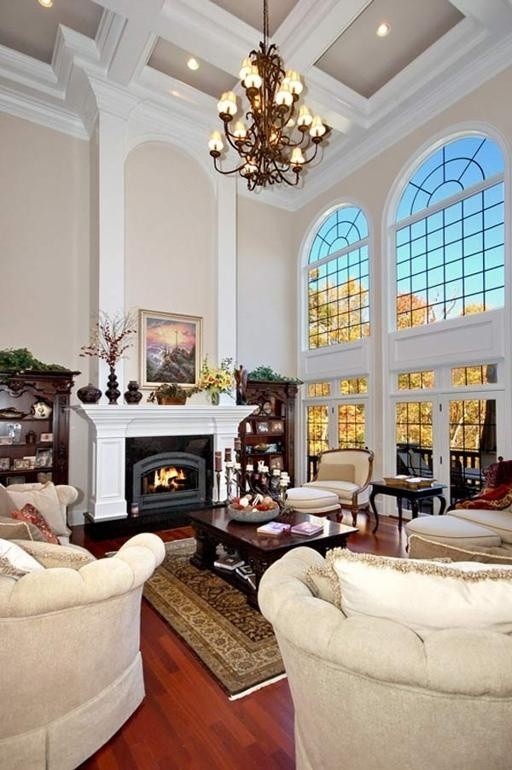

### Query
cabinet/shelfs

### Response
[
  {"left": 237, "top": 379, "right": 304, "bottom": 485},
  {"left": 0, "top": 369, "right": 78, "bottom": 485}
]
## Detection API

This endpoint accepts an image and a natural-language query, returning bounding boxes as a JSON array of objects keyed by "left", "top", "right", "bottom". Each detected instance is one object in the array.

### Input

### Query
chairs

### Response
[{"left": 0, "top": 484, "right": 166, "bottom": 770}]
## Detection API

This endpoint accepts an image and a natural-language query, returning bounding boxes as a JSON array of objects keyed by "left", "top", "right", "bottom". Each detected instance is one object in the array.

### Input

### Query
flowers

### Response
[{"left": 191, "top": 354, "right": 238, "bottom": 400}]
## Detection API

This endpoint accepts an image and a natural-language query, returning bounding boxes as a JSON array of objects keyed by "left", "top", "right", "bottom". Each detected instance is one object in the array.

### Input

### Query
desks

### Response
[{"left": 189, "top": 505, "right": 360, "bottom": 616}]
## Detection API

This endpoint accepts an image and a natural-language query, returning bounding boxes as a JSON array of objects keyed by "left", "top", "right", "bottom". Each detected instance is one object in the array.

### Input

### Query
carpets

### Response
[{"left": 104, "top": 537, "right": 288, "bottom": 702}]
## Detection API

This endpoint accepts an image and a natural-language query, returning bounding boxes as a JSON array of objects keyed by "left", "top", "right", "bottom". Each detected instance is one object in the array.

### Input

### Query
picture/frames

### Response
[{"left": 138, "top": 307, "right": 204, "bottom": 390}]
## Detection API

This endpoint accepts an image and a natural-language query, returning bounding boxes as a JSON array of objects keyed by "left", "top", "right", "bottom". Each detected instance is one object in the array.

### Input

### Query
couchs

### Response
[
  {"left": 445, "top": 458, "right": 510, "bottom": 554},
  {"left": 299, "top": 448, "right": 375, "bottom": 527},
  {"left": 256, "top": 545, "right": 512, "bottom": 770}
]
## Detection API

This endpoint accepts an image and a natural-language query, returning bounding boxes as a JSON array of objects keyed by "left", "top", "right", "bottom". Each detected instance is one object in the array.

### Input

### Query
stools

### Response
[
  {"left": 279, "top": 487, "right": 344, "bottom": 527},
  {"left": 405, "top": 515, "right": 504, "bottom": 564}
]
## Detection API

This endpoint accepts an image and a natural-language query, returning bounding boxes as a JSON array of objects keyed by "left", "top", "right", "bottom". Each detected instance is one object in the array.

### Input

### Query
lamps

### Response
[{"left": 208, "top": 0, "right": 327, "bottom": 192}]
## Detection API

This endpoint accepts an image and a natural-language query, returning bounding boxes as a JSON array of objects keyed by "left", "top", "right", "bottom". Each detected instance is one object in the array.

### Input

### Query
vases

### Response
[
  {"left": 211, "top": 391, "right": 220, "bottom": 406},
  {"left": 76, "top": 383, "right": 102, "bottom": 404},
  {"left": 124, "top": 380, "right": 142, "bottom": 405}
]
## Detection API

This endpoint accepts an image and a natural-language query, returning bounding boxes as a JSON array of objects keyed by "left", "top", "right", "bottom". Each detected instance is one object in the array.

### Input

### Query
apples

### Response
[
  {"left": 268, "top": 502, "right": 274, "bottom": 510},
  {"left": 256, "top": 504, "right": 268, "bottom": 511}
]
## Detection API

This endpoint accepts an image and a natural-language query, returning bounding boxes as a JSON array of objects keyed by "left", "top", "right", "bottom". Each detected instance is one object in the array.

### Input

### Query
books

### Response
[
  {"left": 257, "top": 521, "right": 291, "bottom": 537},
  {"left": 213, "top": 555, "right": 257, "bottom": 591},
  {"left": 291, "top": 521, "right": 324, "bottom": 537}
]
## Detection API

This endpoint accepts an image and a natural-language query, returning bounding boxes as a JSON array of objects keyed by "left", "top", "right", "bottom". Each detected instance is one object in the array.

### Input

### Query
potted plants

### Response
[
  {"left": 78, "top": 311, "right": 140, "bottom": 405},
  {"left": 147, "top": 377, "right": 198, "bottom": 405}
]
